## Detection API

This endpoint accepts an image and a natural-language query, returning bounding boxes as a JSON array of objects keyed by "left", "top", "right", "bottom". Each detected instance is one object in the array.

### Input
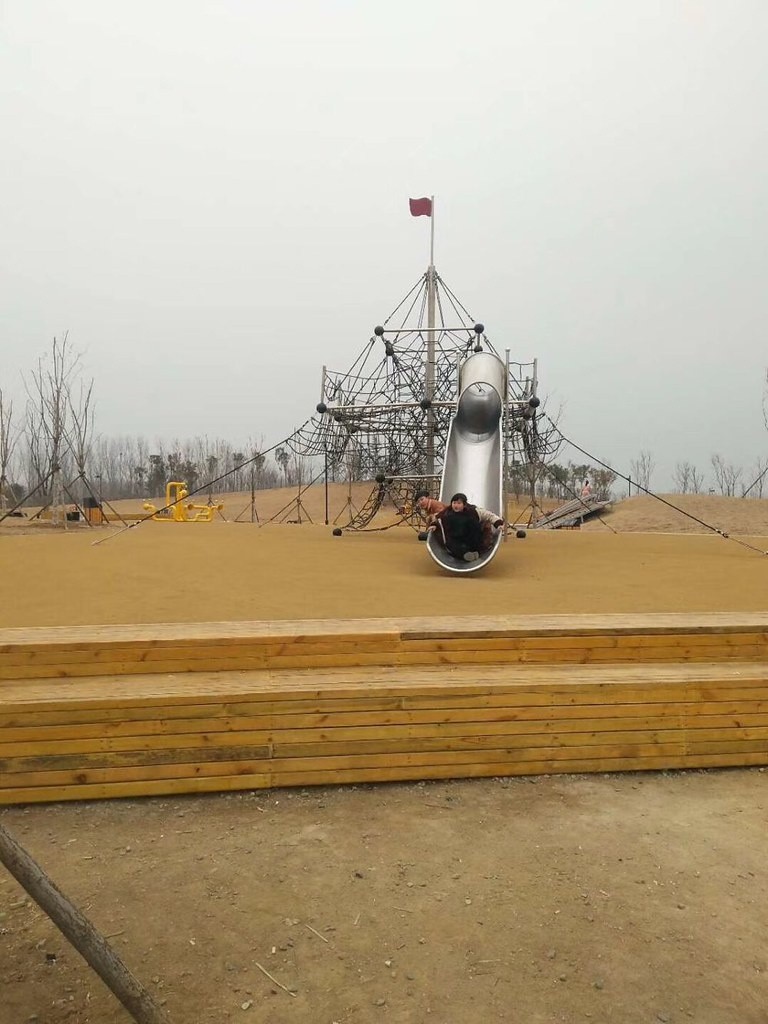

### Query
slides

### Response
[{"left": 426, "top": 352, "right": 506, "bottom": 574}]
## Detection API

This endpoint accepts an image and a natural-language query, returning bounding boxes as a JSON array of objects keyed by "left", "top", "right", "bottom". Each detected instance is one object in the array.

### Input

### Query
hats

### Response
[{"left": 414, "top": 490, "right": 429, "bottom": 502}]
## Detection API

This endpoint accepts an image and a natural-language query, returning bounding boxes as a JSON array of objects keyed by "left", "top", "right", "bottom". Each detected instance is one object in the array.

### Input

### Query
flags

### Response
[{"left": 409, "top": 198, "right": 432, "bottom": 216}]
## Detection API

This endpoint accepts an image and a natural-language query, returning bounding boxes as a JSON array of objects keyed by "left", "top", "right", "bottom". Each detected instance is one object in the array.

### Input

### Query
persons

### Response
[
  {"left": 414, "top": 490, "right": 492, "bottom": 551},
  {"left": 424, "top": 493, "right": 505, "bottom": 561},
  {"left": 582, "top": 480, "right": 591, "bottom": 497}
]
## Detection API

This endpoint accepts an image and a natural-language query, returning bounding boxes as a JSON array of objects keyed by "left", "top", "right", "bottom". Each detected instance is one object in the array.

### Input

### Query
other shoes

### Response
[{"left": 463, "top": 551, "right": 479, "bottom": 561}]
